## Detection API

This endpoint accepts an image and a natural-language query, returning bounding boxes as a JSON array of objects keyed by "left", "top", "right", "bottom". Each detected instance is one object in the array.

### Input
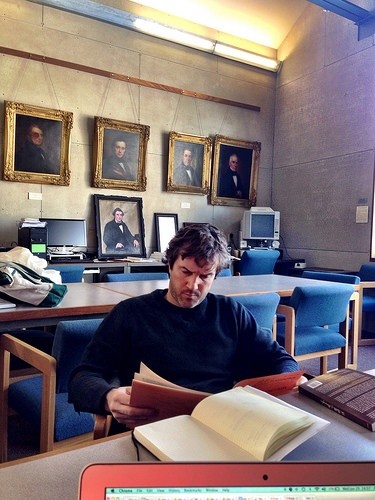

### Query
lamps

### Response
[{"left": 128, "top": 16, "right": 280, "bottom": 71}]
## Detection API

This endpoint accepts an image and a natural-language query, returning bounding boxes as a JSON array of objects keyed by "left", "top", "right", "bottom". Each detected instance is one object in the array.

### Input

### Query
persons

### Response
[{"left": 66, "top": 222, "right": 308, "bottom": 436}]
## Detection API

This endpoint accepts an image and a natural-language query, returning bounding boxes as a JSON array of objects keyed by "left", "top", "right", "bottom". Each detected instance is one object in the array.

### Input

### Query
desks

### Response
[
  {"left": 0, "top": 281, "right": 133, "bottom": 334},
  {"left": 49, "top": 257, "right": 162, "bottom": 274},
  {"left": 0, "top": 369, "right": 375, "bottom": 500},
  {"left": 91, "top": 274, "right": 360, "bottom": 368}
]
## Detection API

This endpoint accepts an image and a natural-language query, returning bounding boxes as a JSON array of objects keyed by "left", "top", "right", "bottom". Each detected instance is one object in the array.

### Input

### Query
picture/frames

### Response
[
  {"left": 167, "top": 132, "right": 213, "bottom": 196},
  {"left": 93, "top": 193, "right": 146, "bottom": 259},
  {"left": 3, "top": 100, "right": 74, "bottom": 186},
  {"left": 208, "top": 134, "right": 262, "bottom": 207},
  {"left": 91, "top": 115, "right": 150, "bottom": 191}
]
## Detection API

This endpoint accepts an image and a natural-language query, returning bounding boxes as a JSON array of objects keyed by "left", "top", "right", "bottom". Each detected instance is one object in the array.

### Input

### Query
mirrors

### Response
[{"left": 155, "top": 212, "right": 179, "bottom": 253}]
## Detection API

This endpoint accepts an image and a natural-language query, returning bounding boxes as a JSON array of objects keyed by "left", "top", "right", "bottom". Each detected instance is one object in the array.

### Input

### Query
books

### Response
[
  {"left": 298, "top": 368, "right": 375, "bottom": 432},
  {"left": 133, "top": 385, "right": 331, "bottom": 462},
  {"left": 128, "top": 362, "right": 304, "bottom": 431},
  {"left": 0, "top": 298, "right": 16, "bottom": 308}
]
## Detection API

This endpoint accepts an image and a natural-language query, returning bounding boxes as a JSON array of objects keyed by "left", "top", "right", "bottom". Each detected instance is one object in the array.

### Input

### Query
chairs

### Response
[{"left": 0, "top": 249, "right": 375, "bottom": 464}]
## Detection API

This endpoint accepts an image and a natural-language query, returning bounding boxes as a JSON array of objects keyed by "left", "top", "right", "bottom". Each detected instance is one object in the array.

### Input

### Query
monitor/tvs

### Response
[
  {"left": 244, "top": 211, "right": 279, "bottom": 240},
  {"left": 39, "top": 218, "right": 87, "bottom": 254},
  {"left": 78, "top": 460, "right": 375, "bottom": 492}
]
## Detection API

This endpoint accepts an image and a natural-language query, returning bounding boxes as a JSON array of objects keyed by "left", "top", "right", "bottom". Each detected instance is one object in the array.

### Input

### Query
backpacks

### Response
[{"left": 0, "top": 259, "right": 68, "bottom": 308}]
